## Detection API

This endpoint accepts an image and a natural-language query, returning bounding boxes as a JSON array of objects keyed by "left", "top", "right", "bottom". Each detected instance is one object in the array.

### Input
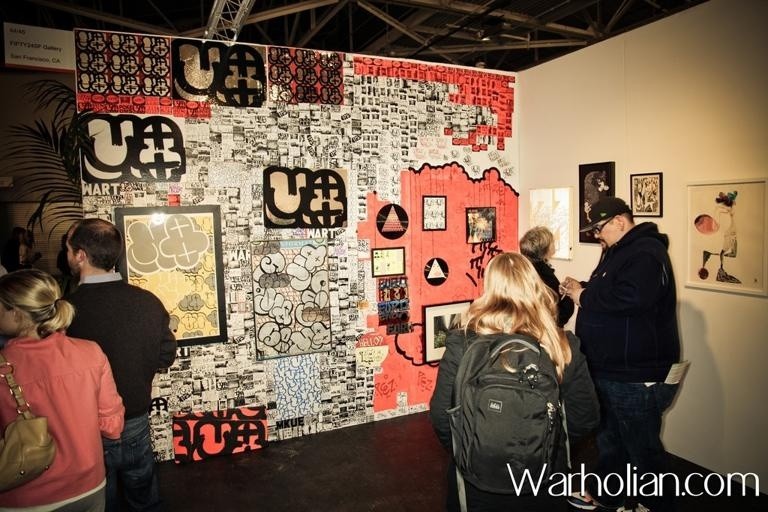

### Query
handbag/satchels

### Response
[{"left": 0, "top": 414, "right": 56, "bottom": 494}]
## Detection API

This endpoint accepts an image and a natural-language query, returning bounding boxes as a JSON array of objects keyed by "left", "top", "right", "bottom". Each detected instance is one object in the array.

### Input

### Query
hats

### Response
[{"left": 576, "top": 198, "right": 632, "bottom": 233}]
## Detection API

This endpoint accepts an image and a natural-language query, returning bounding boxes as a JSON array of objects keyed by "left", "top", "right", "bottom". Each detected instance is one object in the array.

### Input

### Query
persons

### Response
[
  {"left": 558, "top": 196, "right": 681, "bottom": 512},
  {"left": 428, "top": 252, "right": 602, "bottom": 512},
  {"left": 0, "top": 267, "right": 126, "bottom": 512},
  {"left": 61, "top": 217, "right": 177, "bottom": 512},
  {"left": 519, "top": 226, "right": 575, "bottom": 330}
]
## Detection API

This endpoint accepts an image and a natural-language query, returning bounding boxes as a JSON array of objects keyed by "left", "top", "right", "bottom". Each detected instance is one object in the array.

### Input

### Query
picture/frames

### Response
[
  {"left": 683, "top": 177, "right": 768, "bottom": 298},
  {"left": 630, "top": 172, "right": 664, "bottom": 218},
  {"left": 371, "top": 246, "right": 405, "bottom": 278},
  {"left": 422, "top": 195, "right": 447, "bottom": 231},
  {"left": 578, "top": 161, "right": 616, "bottom": 243},
  {"left": 113, "top": 204, "right": 228, "bottom": 349},
  {"left": 465, "top": 206, "right": 497, "bottom": 244},
  {"left": 422, "top": 299, "right": 476, "bottom": 367}
]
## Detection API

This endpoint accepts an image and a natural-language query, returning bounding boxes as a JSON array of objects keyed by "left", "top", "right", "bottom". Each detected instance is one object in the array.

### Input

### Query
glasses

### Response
[{"left": 588, "top": 215, "right": 616, "bottom": 234}]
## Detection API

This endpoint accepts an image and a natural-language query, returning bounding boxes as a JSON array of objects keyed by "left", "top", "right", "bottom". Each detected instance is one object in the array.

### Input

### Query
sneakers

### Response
[
  {"left": 568, "top": 488, "right": 626, "bottom": 512},
  {"left": 615, "top": 500, "right": 661, "bottom": 512}
]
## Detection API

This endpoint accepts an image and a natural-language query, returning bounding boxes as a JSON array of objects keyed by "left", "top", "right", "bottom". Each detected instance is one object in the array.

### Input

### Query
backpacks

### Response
[{"left": 443, "top": 330, "right": 565, "bottom": 496}]
